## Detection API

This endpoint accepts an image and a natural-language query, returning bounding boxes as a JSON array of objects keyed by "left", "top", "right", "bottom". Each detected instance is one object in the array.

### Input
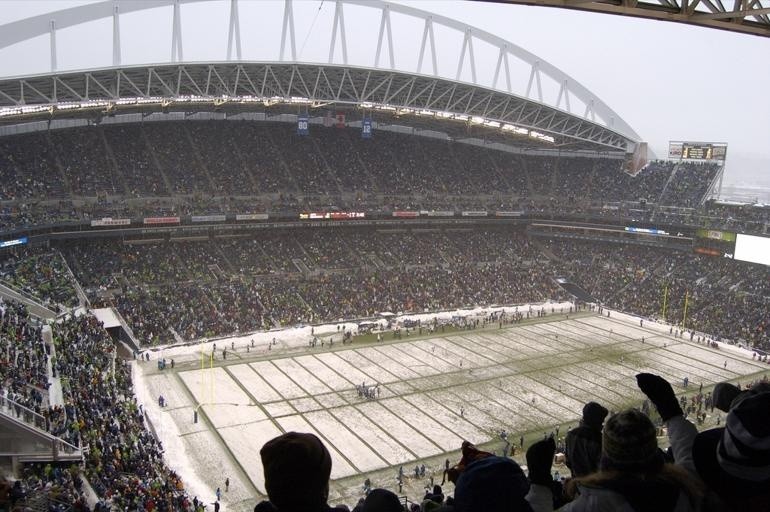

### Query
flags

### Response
[
  {"left": 322, "top": 109, "right": 333, "bottom": 129},
  {"left": 362, "top": 118, "right": 373, "bottom": 138},
  {"left": 335, "top": 111, "right": 345, "bottom": 128},
  {"left": 297, "top": 115, "right": 308, "bottom": 136}
]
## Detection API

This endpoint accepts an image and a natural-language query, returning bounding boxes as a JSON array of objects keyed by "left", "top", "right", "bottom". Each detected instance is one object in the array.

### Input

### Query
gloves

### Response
[
  {"left": 527, "top": 437, "right": 554, "bottom": 484},
  {"left": 636, "top": 373, "right": 683, "bottom": 420}
]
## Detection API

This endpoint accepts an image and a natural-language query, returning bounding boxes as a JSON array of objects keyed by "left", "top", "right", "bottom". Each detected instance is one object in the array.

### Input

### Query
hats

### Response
[
  {"left": 603, "top": 409, "right": 657, "bottom": 459},
  {"left": 363, "top": 488, "right": 405, "bottom": 511},
  {"left": 260, "top": 433, "right": 331, "bottom": 510},
  {"left": 696, "top": 389, "right": 769, "bottom": 496},
  {"left": 583, "top": 402, "right": 608, "bottom": 424},
  {"left": 456, "top": 456, "right": 530, "bottom": 505}
]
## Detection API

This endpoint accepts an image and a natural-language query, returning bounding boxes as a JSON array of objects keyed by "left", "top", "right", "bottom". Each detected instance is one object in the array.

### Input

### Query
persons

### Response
[{"left": 0, "top": 119, "right": 769, "bottom": 512}]
